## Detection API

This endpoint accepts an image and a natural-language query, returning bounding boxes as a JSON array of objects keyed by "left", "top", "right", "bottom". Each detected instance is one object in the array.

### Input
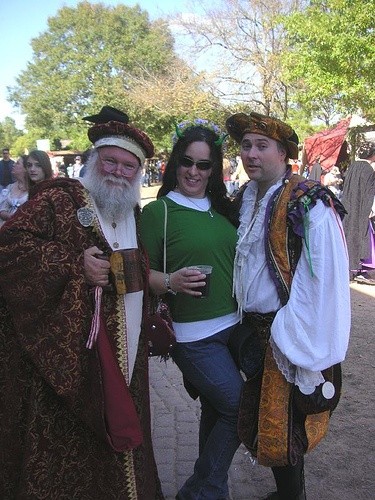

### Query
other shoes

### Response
[{"left": 356, "top": 272, "right": 375, "bottom": 285}]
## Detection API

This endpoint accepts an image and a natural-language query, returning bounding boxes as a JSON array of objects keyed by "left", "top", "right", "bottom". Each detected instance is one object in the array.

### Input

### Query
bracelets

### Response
[{"left": 164, "top": 273, "right": 177, "bottom": 295}]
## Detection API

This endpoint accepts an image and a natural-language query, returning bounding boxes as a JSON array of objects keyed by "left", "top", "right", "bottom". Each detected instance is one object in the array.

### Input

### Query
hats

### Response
[
  {"left": 82, "top": 105, "right": 129, "bottom": 126},
  {"left": 226, "top": 112, "right": 299, "bottom": 160},
  {"left": 88, "top": 120, "right": 155, "bottom": 169},
  {"left": 357, "top": 142, "right": 375, "bottom": 159}
]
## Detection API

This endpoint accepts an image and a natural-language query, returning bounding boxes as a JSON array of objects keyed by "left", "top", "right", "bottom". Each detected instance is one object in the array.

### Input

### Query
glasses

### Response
[
  {"left": 97, "top": 152, "right": 140, "bottom": 178},
  {"left": 179, "top": 155, "right": 214, "bottom": 170}
]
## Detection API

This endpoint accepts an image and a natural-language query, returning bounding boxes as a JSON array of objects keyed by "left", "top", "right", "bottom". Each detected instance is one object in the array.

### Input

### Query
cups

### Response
[
  {"left": 187, "top": 266, "right": 212, "bottom": 299},
  {"left": 94, "top": 248, "right": 144, "bottom": 294}
]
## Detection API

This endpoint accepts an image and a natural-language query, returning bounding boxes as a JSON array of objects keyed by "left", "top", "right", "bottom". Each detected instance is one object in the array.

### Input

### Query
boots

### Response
[{"left": 263, "top": 455, "right": 307, "bottom": 500}]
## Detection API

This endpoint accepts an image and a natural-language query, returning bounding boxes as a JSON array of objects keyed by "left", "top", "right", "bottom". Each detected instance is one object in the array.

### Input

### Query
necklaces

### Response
[
  {"left": 18, "top": 181, "right": 25, "bottom": 192},
  {"left": 179, "top": 190, "right": 213, "bottom": 217}
]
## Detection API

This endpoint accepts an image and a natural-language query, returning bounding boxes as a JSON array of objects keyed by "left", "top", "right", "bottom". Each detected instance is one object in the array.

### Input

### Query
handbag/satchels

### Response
[{"left": 140, "top": 301, "right": 177, "bottom": 363}]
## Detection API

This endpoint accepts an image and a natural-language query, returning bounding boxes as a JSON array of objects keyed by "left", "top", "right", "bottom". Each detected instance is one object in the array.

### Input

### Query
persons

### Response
[
  {"left": 225, "top": 112, "right": 352, "bottom": 500},
  {"left": 0, "top": 141, "right": 375, "bottom": 286},
  {"left": 0, "top": 121, "right": 162, "bottom": 500},
  {"left": 138, "top": 118, "right": 246, "bottom": 500}
]
